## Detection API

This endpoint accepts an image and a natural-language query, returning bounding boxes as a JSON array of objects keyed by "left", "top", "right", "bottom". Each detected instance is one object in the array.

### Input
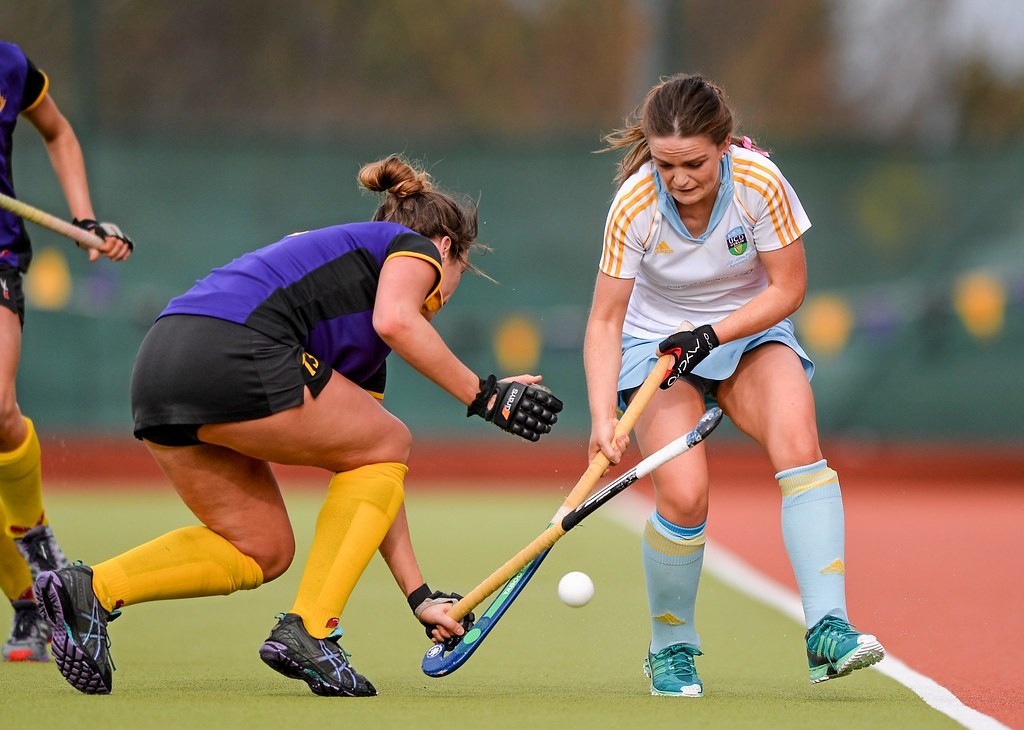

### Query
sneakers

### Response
[
  {"left": 34, "top": 559, "right": 124, "bottom": 695},
  {"left": 643, "top": 639, "right": 704, "bottom": 697},
  {"left": 12, "top": 525, "right": 73, "bottom": 582},
  {"left": 260, "top": 613, "right": 376, "bottom": 698},
  {"left": 804, "top": 613, "right": 886, "bottom": 685},
  {"left": 3, "top": 600, "right": 53, "bottom": 662}
]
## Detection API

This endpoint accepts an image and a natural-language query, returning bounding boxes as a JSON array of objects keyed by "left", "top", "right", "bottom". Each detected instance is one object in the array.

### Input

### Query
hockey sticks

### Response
[
  {"left": 0, "top": 192, "right": 106, "bottom": 254},
  {"left": 421, "top": 354, "right": 672, "bottom": 678},
  {"left": 425, "top": 406, "right": 724, "bottom": 641}
]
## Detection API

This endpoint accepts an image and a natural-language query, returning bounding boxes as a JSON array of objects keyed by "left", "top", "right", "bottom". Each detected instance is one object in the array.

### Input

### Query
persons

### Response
[
  {"left": 32, "top": 152, "right": 565, "bottom": 697},
  {"left": 0, "top": 43, "right": 135, "bottom": 658},
  {"left": 584, "top": 72, "right": 884, "bottom": 700}
]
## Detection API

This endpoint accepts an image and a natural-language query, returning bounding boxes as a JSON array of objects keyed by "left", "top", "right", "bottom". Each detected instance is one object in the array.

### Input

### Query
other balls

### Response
[{"left": 558, "top": 571, "right": 595, "bottom": 608}]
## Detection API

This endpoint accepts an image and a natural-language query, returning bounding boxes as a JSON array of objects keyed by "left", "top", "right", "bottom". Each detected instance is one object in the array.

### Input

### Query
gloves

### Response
[{"left": 655, "top": 321, "right": 721, "bottom": 390}]
